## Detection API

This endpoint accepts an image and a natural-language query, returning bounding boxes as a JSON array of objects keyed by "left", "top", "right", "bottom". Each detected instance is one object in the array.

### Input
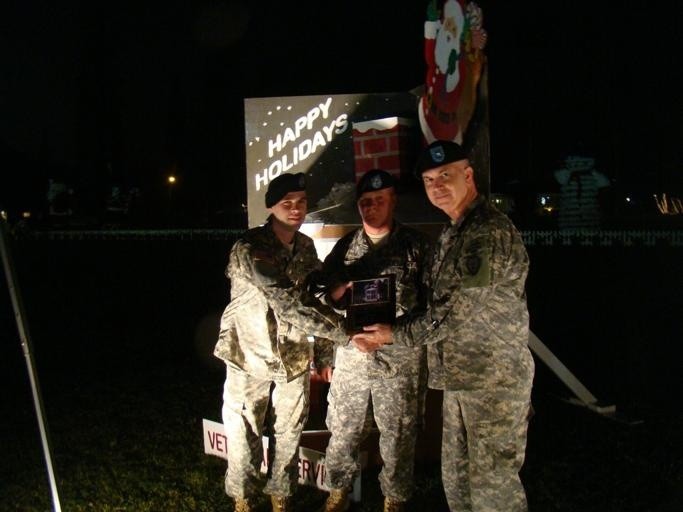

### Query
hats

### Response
[
  {"left": 356, "top": 168, "right": 396, "bottom": 198},
  {"left": 414, "top": 140, "right": 468, "bottom": 180},
  {"left": 265, "top": 172, "right": 306, "bottom": 208}
]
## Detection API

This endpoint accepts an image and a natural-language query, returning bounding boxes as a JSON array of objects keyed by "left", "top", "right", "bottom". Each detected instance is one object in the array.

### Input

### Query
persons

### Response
[
  {"left": 213, "top": 172, "right": 382, "bottom": 511},
  {"left": 352, "top": 138, "right": 533, "bottom": 511},
  {"left": 305, "top": 170, "right": 435, "bottom": 511}
]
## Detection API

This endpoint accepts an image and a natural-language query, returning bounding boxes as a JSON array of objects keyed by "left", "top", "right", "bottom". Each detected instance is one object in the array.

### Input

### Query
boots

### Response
[
  {"left": 235, "top": 497, "right": 254, "bottom": 512},
  {"left": 384, "top": 496, "right": 402, "bottom": 512},
  {"left": 271, "top": 495, "right": 290, "bottom": 512},
  {"left": 319, "top": 490, "right": 350, "bottom": 512}
]
